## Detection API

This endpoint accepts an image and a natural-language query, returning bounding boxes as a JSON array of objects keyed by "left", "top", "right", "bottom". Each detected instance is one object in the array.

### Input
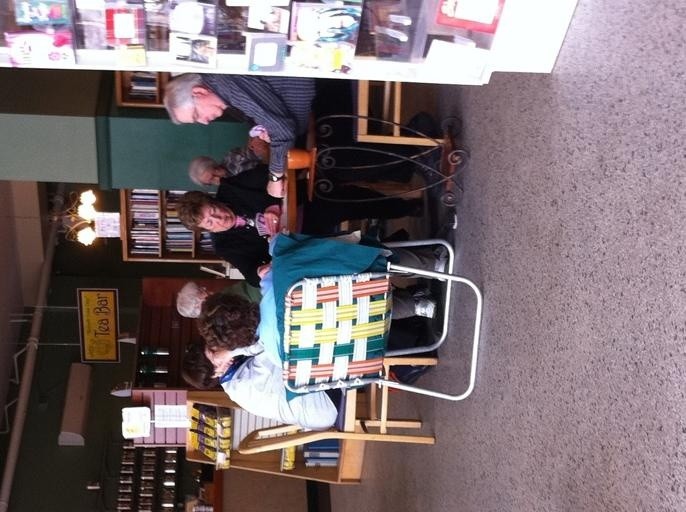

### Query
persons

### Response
[{"left": 118, "top": 76, "right": 455, "bottom": 437}]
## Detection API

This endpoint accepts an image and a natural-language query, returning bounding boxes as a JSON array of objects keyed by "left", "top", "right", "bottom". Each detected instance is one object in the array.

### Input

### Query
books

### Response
[{"left": 296, "top": 442, "right": 345, "bottom": 469}]
